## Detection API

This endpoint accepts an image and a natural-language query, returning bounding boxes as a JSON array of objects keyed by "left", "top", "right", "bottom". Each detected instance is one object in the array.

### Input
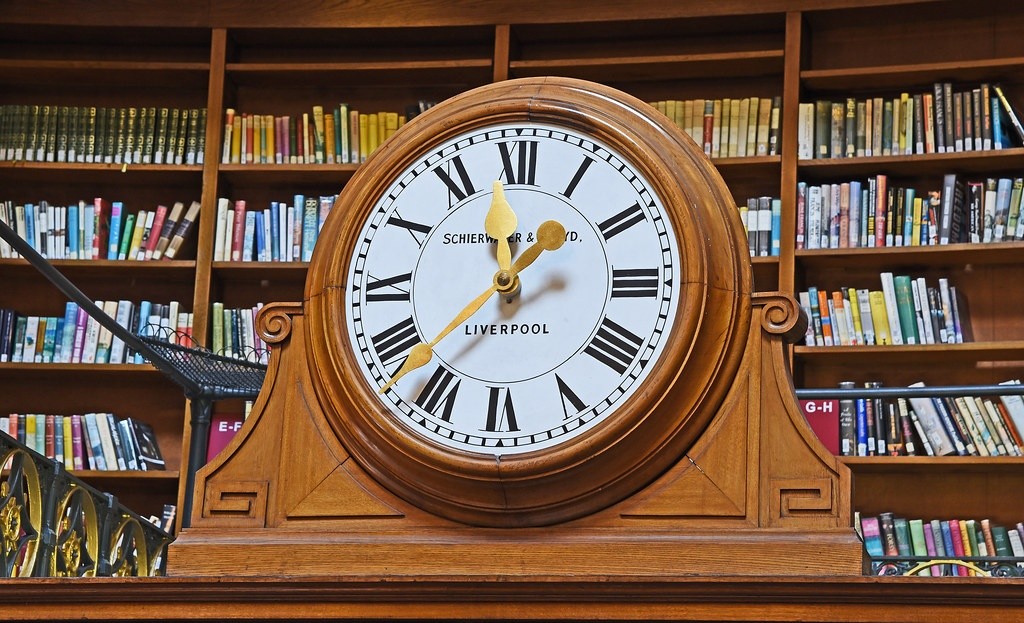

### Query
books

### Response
[
  {"left": 0, "top": 105, "right": 406, "bottom": 472},
  {"left": 644, "top": 83, "right": 1022, "bottom": 578}
]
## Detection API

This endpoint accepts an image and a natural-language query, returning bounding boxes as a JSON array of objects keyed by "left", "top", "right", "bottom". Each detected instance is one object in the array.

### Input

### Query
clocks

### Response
[{"left": 302, "top": 76, "right": 754, "bottom": 529}]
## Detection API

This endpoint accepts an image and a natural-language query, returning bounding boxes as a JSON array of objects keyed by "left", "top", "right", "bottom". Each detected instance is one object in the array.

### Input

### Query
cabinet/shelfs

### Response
[{"left": 0, "top": 0, "right": 1024, "bottom": 575}]
